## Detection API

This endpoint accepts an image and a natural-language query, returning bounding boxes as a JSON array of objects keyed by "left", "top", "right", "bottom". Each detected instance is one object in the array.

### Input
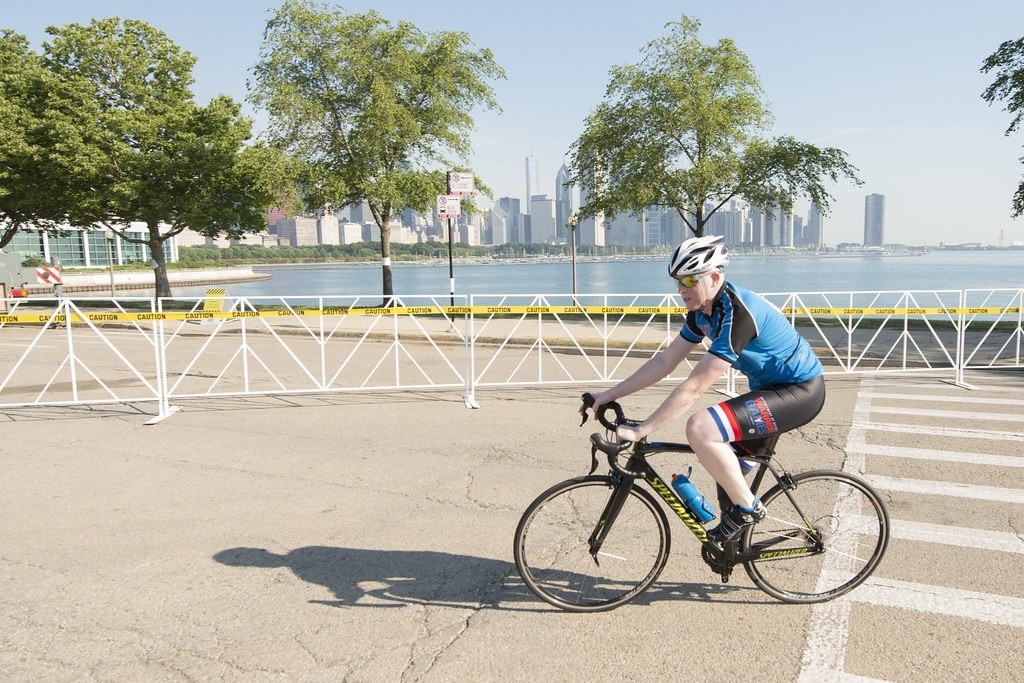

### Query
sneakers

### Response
[{"left": 706, "top": 495, "right": 767, "bottom": 542}]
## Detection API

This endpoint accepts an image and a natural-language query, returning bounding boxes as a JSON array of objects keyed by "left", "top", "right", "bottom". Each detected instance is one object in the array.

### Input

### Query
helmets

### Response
[{"left": 667, "top": 235, "right": 730, "bottom": 277}]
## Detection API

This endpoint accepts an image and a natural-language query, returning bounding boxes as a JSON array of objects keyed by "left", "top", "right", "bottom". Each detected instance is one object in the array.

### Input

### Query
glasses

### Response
[{"left": 672, "top": 269, "right": 725, "bottom": 288}]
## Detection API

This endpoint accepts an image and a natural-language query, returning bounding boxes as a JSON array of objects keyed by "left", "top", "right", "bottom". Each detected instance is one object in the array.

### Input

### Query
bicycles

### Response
[{"left": 512, "top": 392, "right": 888, "bottom": 615}]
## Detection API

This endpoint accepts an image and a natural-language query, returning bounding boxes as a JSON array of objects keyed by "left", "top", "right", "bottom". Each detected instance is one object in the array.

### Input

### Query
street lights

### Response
[
  {"left": 105, "top": 228, "right": 117, "bottom": 297},
  {"left": 565, "top": 217, "right": 578, "bottom": 306}
]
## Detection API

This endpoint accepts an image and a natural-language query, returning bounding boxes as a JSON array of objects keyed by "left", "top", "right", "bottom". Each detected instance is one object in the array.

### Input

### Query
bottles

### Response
[{"left": 671, "top": 466, "right": 716, "bottom": 524}]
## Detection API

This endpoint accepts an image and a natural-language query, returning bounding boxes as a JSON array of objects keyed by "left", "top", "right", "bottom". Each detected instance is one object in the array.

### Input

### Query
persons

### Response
[{"left": 578, "top": 234, "right": 826, "bottom": 542}]
